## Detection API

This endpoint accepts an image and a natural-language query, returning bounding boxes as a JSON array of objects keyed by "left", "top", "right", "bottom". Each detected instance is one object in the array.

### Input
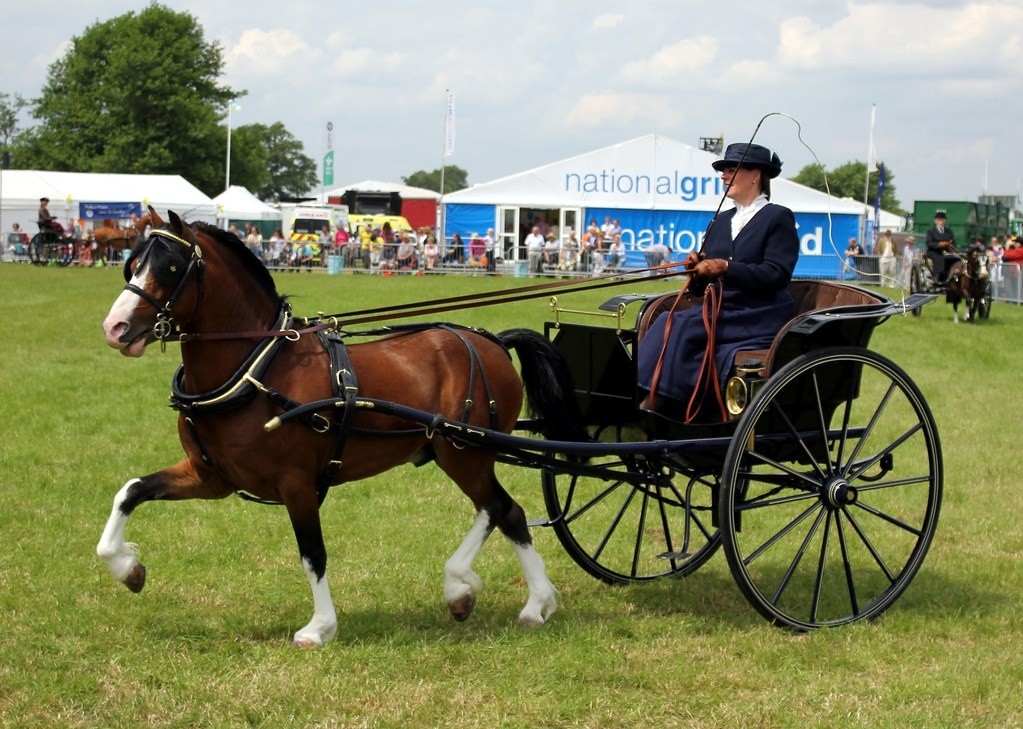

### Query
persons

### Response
[
  {"left": 128, "top": 213, "right": 138, "bottom": 228},
  {"left": 926, "top": 212, "right": 956, "bottom": 284},
  {"left": 584, "top": 218, "right": 626, "bottom": 274},
  {"left": 520, "top": 216, "right": 579, "bottom": 279},
  {"left": 878, "top": 230, "right": 898, "bottom": 287},
  {"left": 469, "top": 229, "right": 501, "bottom": 276},
  {"left": 231, "top": 223, "right": 313, "bottom": 272},
  {"left": 644, "top": 245, "right": 673, "bottom": 275},
  {"left": 319, "top": 222, "right": 438, "bottom": 274},
  {"left": 844, "top": 238, "right": 865, "bottom": 278},
  {"left": 67, "top": 218, "right": 98, "bottom": 259},
  {"left": 899, "top": 237, "right": 921, "bottom": 289},
  {"left": 977, "top": 236, "right": 1023, "bottom": 305},
  {"left": 444, "top": 234, "right": 465, "bottom": 268},
  {"left": 39, "top": 198, "right": 72, "bottom": 238},
  {"left": 638, "top": 143, "right": 800, "bottom": 421},
  {"left": 8, "top": 224, "right": 26, "bottom": 263}
]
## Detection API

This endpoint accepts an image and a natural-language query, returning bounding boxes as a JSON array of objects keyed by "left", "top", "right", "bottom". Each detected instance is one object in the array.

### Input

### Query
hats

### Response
[
  {"left": 712, "top": 142, "right": 781, "bottom": 178},
  {"left": 935, "top": 211, "right": 948, "bottom": 220}
]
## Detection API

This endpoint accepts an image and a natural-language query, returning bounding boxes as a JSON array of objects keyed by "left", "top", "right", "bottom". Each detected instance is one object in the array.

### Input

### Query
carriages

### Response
[
  {"left": 96, "top": 204, "right": 945, "bottom": 657},
  {"left": 910, "top": 243, "right": 995, "bottom": 324},
  {"left": 28, "top": 213, "right": 151, "bottom": 269}
]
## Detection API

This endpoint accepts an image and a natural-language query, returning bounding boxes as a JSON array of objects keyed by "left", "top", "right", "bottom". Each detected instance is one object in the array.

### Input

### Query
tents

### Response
[
  {"left": 0, "top": 169, "right": 217, "bottom": 260},
  {"left": 216, "top": 187, "right": 283, "bottom": 246},
  {"left": 444, "top": 136, "right": 869, "bottom": 277}
]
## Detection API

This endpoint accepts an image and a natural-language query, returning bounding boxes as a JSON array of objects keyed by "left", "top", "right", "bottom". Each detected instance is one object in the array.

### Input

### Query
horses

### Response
[
  {"left": 948, "top": 254, "right": 990, "bottom": 324},
  {"left": 94, "top": 203, "right": 598, "bottom": 647},
  {"left": 89, "top": 213, "right": 153, "bottom": 268}
]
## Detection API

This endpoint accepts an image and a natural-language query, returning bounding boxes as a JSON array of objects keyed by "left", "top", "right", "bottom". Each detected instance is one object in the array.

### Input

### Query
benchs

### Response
[
  {"left": 635, "top": 280, "right": 892, "bottom": 422},
  {"left": 544, "top": 263, "right": 634, "bottom": 281},
  {"left": 421, "top": 262, "right": 488, "bottom": 274},
  {"left": 37, "top": 221, "right": 53, "bottom": 230},
  {"left": 923, "top": 255, "right": 934, "bottom": 277}
]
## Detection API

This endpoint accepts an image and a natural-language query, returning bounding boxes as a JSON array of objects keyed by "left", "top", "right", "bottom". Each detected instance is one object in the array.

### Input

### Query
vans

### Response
[
  {"left": 290, "top": 206, "right": 336, "bottom": 262},
  {"left": 348, "top": 214, "right": 413, "bottom": 253}
]
